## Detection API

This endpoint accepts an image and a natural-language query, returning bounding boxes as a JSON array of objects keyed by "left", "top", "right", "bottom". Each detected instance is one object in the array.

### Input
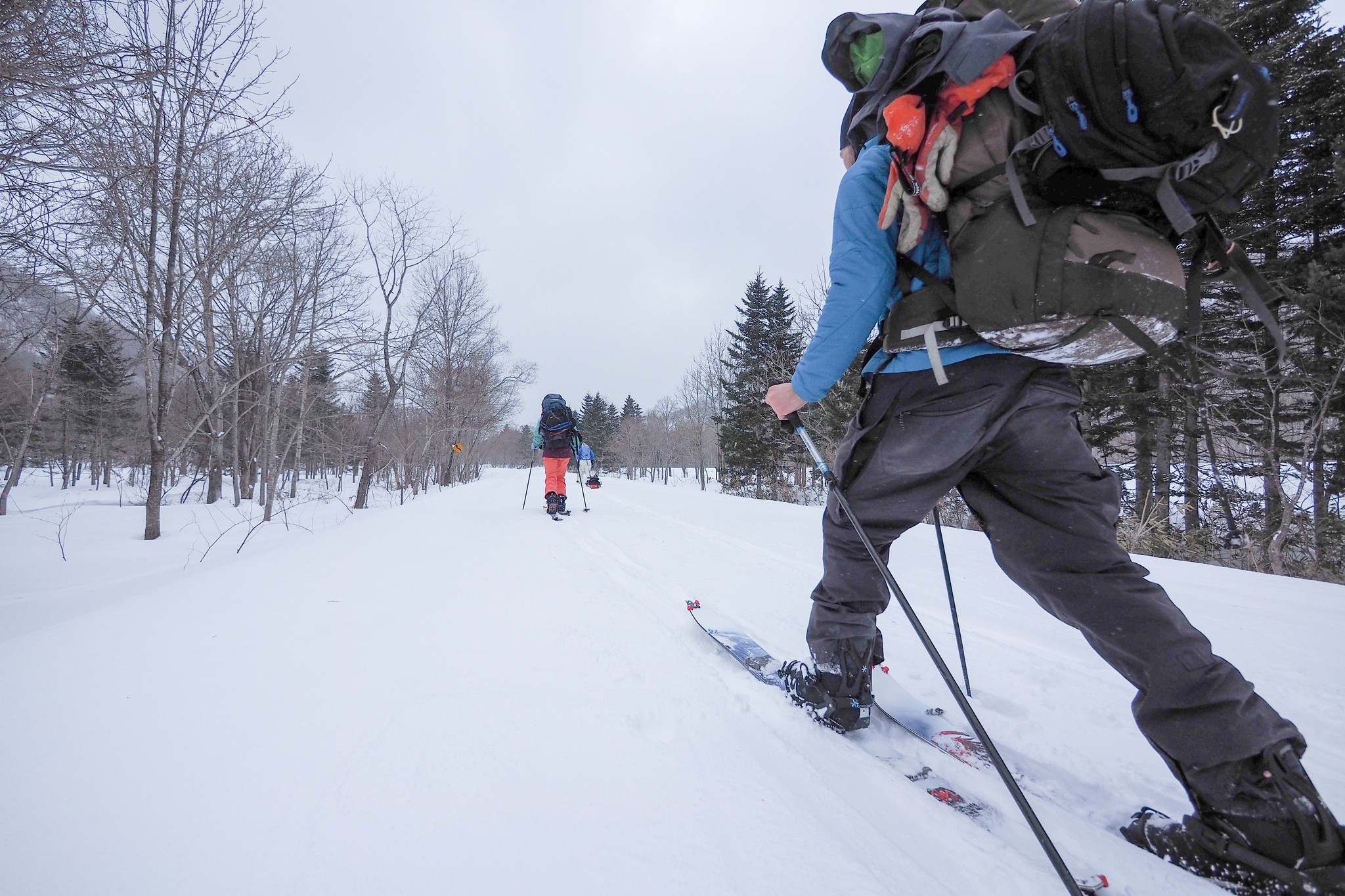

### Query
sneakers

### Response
[
  {"left": 784, "top": 661, "right": 874, "bottom": 731},
  {"left": 1126, "top": 799, "right": 1345, "bottom": 896}
]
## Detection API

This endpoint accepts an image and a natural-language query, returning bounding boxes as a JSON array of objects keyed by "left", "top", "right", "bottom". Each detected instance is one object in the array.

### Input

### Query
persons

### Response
[
  {"left": 577, "top": 442, "right": 594, "bottom": 483},
  {"left": 532, "top": 416, "right": 580, "bottom": 514},
  {"left": 761, "top": 93, "right": 1345, "bottom": 896}
]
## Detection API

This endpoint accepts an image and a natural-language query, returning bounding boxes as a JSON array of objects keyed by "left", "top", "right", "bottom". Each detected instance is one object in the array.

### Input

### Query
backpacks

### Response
[
  {"left": 540, "top": 394, "right": 573, "bottom": 434},
  {"left": 821, "top": 0, "right": 1281, "bottom": 365},
  {"left": 581, "top": 444, "right": 590, "bottom": 456}
]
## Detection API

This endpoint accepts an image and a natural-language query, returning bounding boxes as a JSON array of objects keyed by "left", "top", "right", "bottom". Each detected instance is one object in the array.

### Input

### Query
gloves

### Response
[
  {"left": 531, "top": 443, "right": 539, "bottom": 449},
  {"left": 591, "top": 463, "right": 594, "bottom": 466},
  {"left": 577, "top": 464, "right": 579, "bottom": 469}
]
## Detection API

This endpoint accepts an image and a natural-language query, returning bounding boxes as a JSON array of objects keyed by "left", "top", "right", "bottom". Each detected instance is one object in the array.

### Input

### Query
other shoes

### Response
[
  {"left": 558, "top": 503, "right": 567, "bottom": 512},
  {"left": 547, "top": 495, "right": 557, "bottom": 514}
]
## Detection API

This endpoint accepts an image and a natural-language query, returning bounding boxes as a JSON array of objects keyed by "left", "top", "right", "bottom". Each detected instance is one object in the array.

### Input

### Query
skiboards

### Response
[
  {"left": 682, "top": 597, "right": 1345, "bottom": 895},
  {"left": 544, "top": 504, "right": 571, "bottom": 521}
]
